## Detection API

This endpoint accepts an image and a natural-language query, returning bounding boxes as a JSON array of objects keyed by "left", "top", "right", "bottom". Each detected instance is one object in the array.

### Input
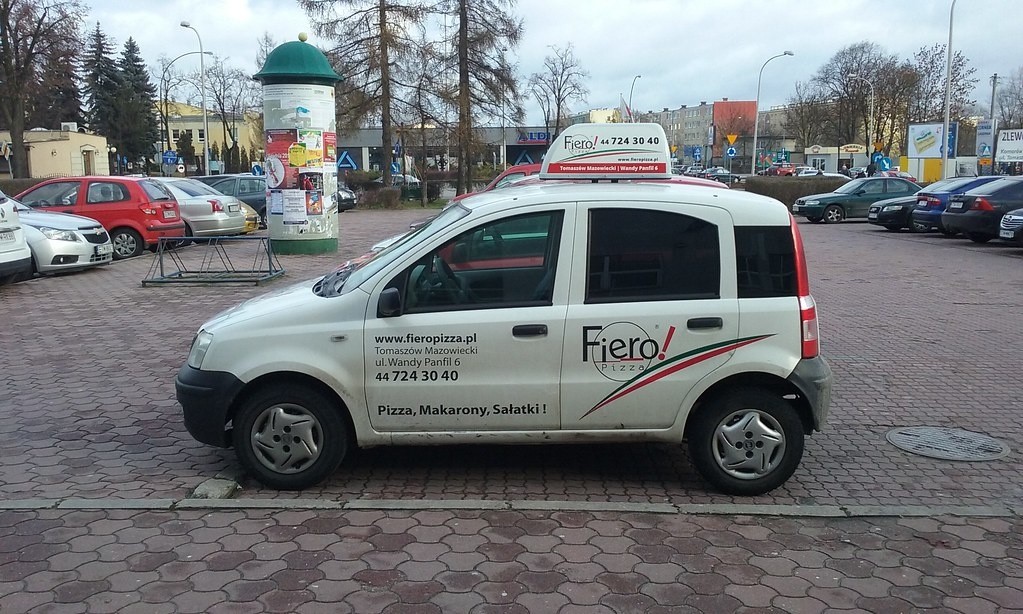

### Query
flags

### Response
[{"left": 619, "top": 96, "right": 632, "bottom": 121}]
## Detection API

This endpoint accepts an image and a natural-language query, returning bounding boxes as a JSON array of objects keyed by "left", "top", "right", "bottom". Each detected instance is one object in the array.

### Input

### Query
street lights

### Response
[
  {"left": 159, "top": 51, "right": 213, "bottom": 179},
  {"left": 178, "top": 21, "right": 213, "bottom": 178},
  {"left": 847, "top": 72, "right": 874, "bottom": 176},
  {"left": 751, "top": 51, "right": 794, "bottom": 177},
  {"left": 629, "top": 74, "right": 641, "bottom": 109}
]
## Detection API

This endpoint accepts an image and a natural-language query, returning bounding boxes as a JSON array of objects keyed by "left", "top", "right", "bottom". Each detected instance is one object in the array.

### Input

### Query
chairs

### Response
[
  {"left": 95, "top": 187, "right": 113, "bottom": 203},
  {"left": 530, "top": 234, "right": 602, "bottom": 300}
]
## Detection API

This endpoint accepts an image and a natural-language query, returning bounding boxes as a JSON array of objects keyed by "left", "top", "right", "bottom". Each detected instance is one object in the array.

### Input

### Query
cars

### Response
[
  {"left": 791, "top": 177, "right": 924, "bottom": 223},
  {"left": 209, "top": 176, "right": 268, "bottom": 231},
  {"left": 939, "top": 175, "right": 1022, "bottom": 246},
  {"left": 757, "top": 160, "right": 917, "bottom": 185},
  {"left": 174, "top": 125, "right": 835, "bottom": 503},
  {"left": 63, "top": 176, "right": 246, "bottom": 249},
  {"left": 4, "top": 196, "right": 114, "bottom": 281},
  {"left": 375, "top": 174, "right": 421, "bottom": 184},
  {"left": 672, "top": 164, "right": 737, "bottom": 189},
  {"left": 11, "top": 177, "right": 185, "bottom": 261},
  {"left": 866, "top": 176, "right": 972, "bottom": 233},
  {"left": 915, "top": 177, "right": 1006, "bottom": 240}
]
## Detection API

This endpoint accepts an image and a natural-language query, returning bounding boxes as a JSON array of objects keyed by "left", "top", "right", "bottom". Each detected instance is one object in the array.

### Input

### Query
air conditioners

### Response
[{"left": 60, "top": 121, "right": 78, "bottom": 132}]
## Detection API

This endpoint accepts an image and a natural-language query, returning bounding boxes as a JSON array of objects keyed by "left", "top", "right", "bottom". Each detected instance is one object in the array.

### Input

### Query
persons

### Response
[{"left": 814, "top": 159, "right": 877, "bottom": 180}]
